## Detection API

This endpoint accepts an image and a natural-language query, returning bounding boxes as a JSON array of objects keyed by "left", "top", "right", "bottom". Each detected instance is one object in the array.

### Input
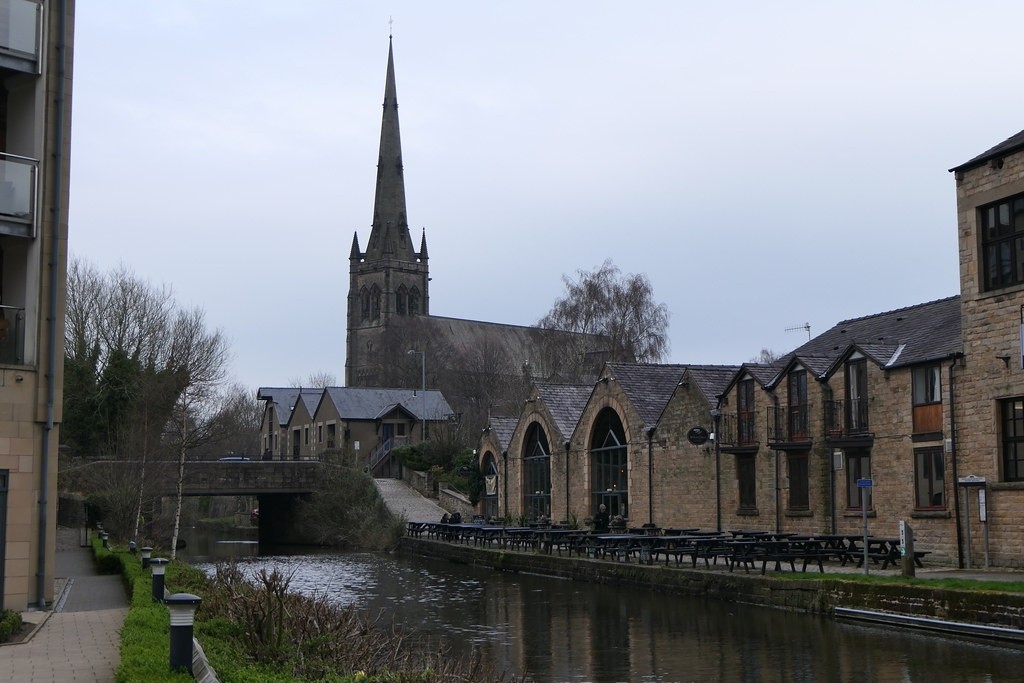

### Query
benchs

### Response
[{"left": 407, "top": 528, "right": 933, "bottom": 574}]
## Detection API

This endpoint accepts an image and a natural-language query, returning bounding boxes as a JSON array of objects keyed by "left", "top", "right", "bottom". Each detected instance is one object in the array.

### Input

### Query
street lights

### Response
[{"left": 407, "top": 350, "right": 425, "bottom": 442}]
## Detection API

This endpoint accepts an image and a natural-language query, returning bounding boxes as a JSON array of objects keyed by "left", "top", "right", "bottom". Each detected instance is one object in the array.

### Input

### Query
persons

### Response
[
  {"left": 441, "top": 512, "right": 449, "bottom": 523},
  {"left": 593, "top": 503, "right": 609, "bottom": 534},
  {"left": 451, "top": 512, "right": 462, "bottom": 524},
  {"left": 610, "top": 513, "right": 626, "bottom": 526}
]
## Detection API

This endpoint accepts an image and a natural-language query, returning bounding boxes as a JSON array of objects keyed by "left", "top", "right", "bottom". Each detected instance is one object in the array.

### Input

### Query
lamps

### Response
[
  {"left": 164, "top": 593, "right": 202, "bottom": 626},
  {"left": 96, "top": 522, "right": 108, "bottom": 539},
  {"left": 150, "top": 558, "right": 168, "bottom": 575},
  {"left": 130, "top": 541, "right": 136, "bottom": 549},
  {"left": 141, "top": 547, "right": 153, "bottom": 558}
]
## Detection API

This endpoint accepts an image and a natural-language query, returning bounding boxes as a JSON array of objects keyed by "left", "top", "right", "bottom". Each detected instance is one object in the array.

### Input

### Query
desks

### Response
[{"left": 409, "top": 520, "right": 917, "bottom": 574}]
